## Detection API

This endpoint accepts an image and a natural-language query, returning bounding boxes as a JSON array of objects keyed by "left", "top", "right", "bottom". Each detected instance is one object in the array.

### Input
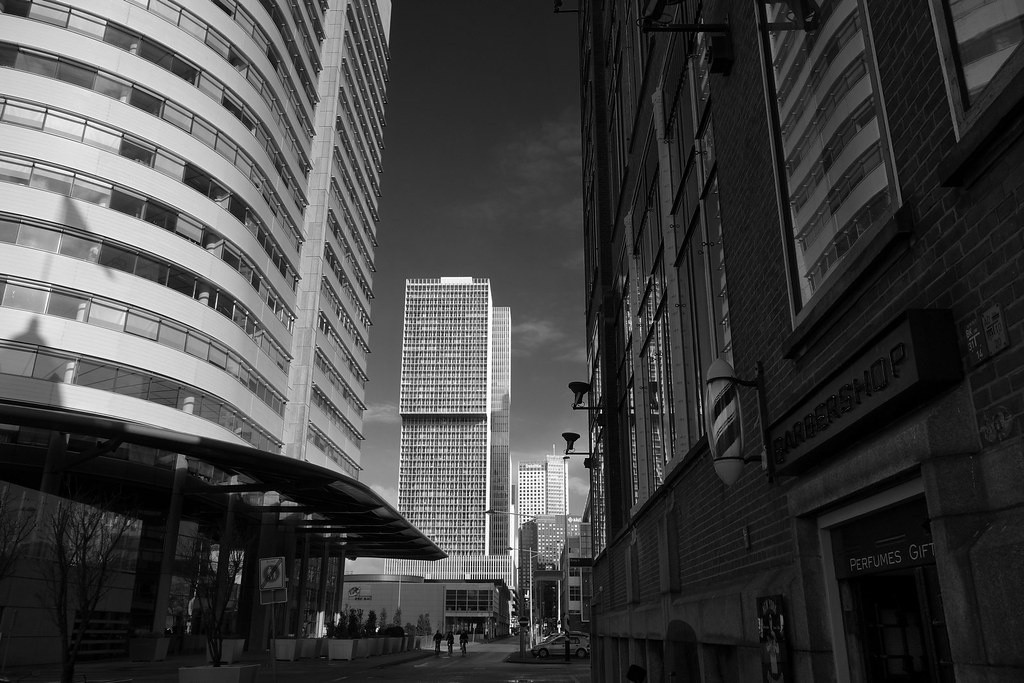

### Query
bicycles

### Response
[
  {"left": 460, "top": 640, "right": 467, "bottom": 657},
  {"left": 435, "top": 639, "right": 439, "bottom": 656},
  {"left": 447, "top": 639, "right": 452, "bottom": 656}
]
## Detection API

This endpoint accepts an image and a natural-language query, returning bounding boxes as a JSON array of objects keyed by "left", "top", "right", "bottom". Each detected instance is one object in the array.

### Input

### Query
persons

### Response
[{"left": 433, "top": 629, "right": 468, "bottom": 654}]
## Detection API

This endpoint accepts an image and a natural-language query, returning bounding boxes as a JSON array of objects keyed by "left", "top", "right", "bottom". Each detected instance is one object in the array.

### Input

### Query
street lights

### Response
[{"left": 504, "top": 547, "right": 542, "bottom": 649}]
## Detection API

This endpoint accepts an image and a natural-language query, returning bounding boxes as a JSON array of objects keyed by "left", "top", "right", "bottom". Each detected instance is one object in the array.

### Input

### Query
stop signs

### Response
[{"left": 518, "top": 617, "right": 528, "bottom": 627}]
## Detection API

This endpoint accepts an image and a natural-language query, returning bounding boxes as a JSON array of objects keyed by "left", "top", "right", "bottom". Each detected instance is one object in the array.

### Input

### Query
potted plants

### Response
[
  {"left": 169, "top": 534, "right": 261, "bottom": 683},
  {"left": 128, "top": 632, "right": 170, "bottom": 661},
  {"left": 270, "top": 604, "right": 434, "bottom": 661},
  {"left": 207, "top": 605, "right": 246, "bottom": 664}
]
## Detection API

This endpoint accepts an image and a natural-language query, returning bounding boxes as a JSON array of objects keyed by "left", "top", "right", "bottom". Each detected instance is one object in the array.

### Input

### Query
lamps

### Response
[
  {"left": 705, "top": 358, "right": 770, "bottom": 487},
  {"left": 562, "top": 433, "right": 590, "bottom": 455},
  {"left": 569, "top": 381, "right": 603, "bottom": 411}
]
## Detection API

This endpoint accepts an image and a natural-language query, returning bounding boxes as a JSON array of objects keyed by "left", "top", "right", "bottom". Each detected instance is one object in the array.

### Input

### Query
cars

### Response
[{"left": 531, "top": 631, "right": 590, "bottom": 659}]
